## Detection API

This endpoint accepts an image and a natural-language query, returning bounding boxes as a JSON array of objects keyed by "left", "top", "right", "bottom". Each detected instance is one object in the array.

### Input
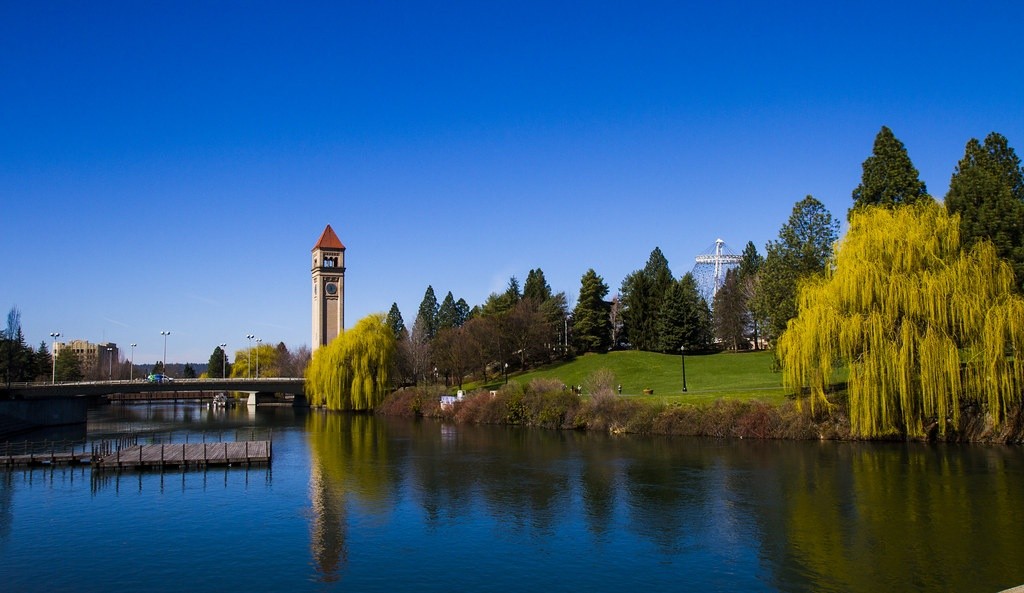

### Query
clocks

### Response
[{"left": 326, "top": 284, "right": 337, "bottom": 293}]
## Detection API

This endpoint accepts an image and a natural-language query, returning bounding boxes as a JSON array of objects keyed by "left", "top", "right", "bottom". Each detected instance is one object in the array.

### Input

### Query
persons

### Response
[
  {"left": 572, "top": 385, "right": 582, "bottom": 397},
  {"left": 618, "top": 384, "right": 622, "bottom": 394}
]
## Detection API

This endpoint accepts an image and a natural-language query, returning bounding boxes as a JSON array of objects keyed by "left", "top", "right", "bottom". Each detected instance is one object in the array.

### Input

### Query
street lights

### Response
[
  {"left": 49, "top": 332, "right": 60, "bottom": 385},
  {"left": 255, "top": 338, "right": 263, "bottom": 377},
  {"left": 160, "top": 330, "right": 171, "bottom": 383},
  {"left": 219, "top": 343, "right": 228, "bottom": 378},
  {"left": 130, "top": 343, "right": 136, "bottom": 381},
  {"left": 433, "top": 366, "right": 438, "bottom": 385},
  {"left": 680, "top": 345, "right": 688, "bottom": 392},
  {"left": 246, "top": 335, "right": 255, "bottom": 378},
  {"left": 504, "top": 362, "right": 509, "bottom": 385}
]
